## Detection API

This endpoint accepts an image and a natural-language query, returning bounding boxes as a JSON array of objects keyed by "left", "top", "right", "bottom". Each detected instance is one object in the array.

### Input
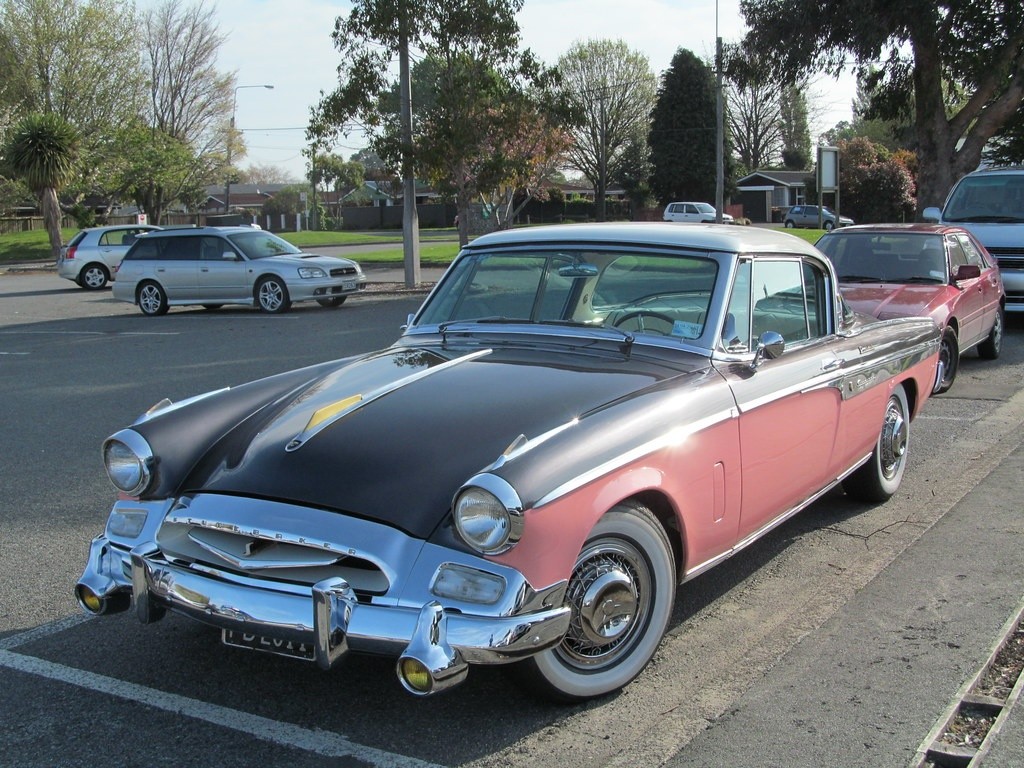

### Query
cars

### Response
[
  {"left": 782, "top": 202, "right": 854, "bottom": 230},
  {"left": 809, "top": 222, "right": 1006, "bottom": 393},
  {"left": 56, "top": 224, "right": 163, "bottom": 289}
]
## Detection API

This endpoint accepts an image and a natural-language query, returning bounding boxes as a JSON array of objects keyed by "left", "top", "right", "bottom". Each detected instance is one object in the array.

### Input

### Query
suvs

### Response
[
  {"left": 113, "top": 222, "right": 367, "bottom": 314},
  {"left": 661, "top": 201, "right": 734, "bottom": 229},
  {"left": 75, "top": 220, "right": 941, "bottom": 704}
]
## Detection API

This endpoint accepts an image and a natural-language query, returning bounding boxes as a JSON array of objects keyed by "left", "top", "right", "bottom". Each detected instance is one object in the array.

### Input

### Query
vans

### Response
[{"left": 920, "top": 165, "right": 1024, "bottom": 312}]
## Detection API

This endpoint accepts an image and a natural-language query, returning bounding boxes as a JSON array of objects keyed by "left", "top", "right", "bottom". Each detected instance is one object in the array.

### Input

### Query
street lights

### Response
[{"left": 222, "top": 81, "right": 276, "bottom": 212}]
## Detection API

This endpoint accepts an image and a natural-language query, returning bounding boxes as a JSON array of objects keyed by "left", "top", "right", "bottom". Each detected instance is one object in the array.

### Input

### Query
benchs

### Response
[{"left": 602, "top": 307, "right": 820, "bottom": 352}]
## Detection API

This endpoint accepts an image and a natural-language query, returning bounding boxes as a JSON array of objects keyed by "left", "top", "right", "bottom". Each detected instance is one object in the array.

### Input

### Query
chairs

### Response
[
  {"left": 122, "top": 233, "right": 135, "bottom": 245},
  {"left": 204, "top": 245, "right": 221, "bottom": 259},
  {"left": 837, "top": 248, "right": 966, "bottom": 280}
]
